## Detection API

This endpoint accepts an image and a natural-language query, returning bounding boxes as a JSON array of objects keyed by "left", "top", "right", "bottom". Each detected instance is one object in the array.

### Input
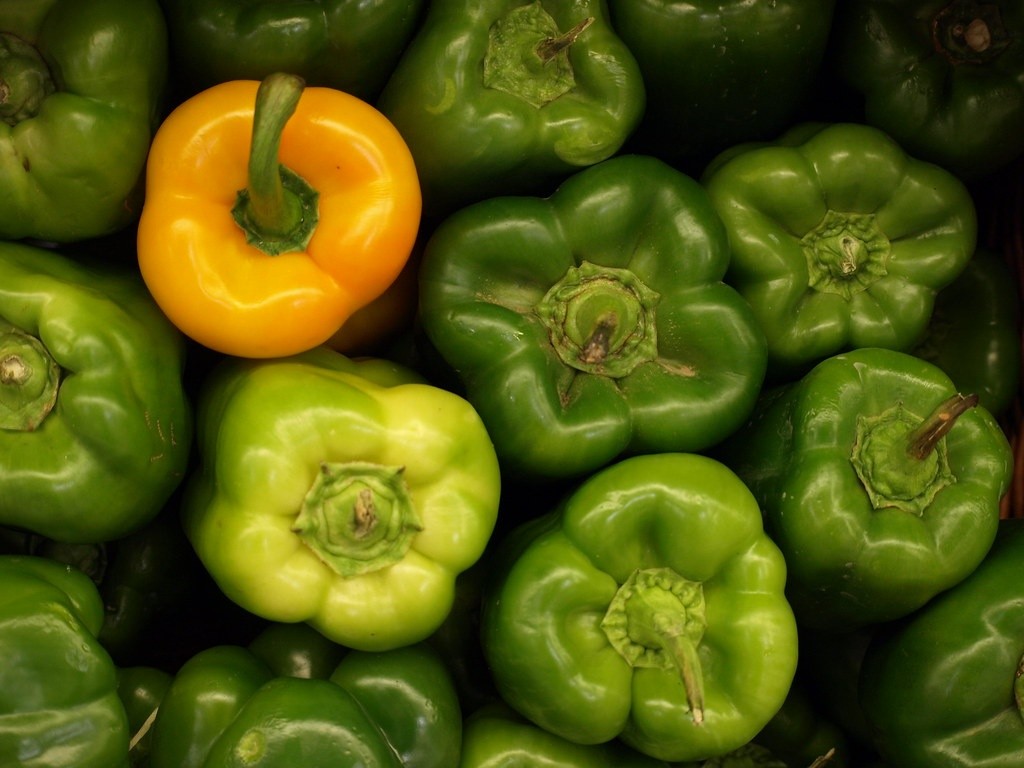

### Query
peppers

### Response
[{"left": 0, "top": 0, "right": 1024, "bottom": 768}]
